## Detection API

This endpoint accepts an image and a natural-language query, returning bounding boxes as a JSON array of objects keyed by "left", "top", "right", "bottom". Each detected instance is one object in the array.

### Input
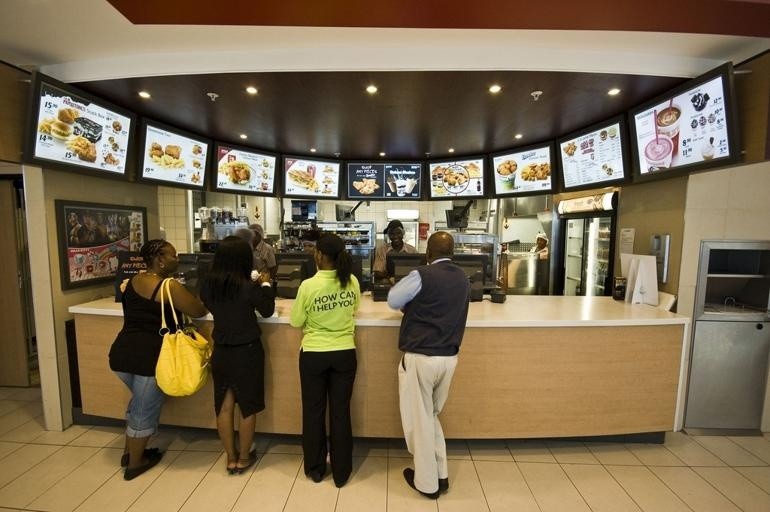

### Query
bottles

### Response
[{"left": 613, "top": 276, "right": 626, "bottom": 300}]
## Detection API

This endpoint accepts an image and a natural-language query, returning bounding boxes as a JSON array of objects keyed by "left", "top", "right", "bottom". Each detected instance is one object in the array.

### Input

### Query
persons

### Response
[
  {"left": 529, "top": 233, "right": 548, "bottom": 261},
  {"left": 302, "top": 221, "right": 321, "bottom": 276},
  {"left": 248, "top": 224, "right": 277, "bottom": 297},
  {"left": 387, "top": 231, "right": 469, "bottom": 500},
  {"left": 108, "top": 239, "right": 209, "bottom": 480},
  {"left": 290, "top": 234, "right": 360, "bottom": 487},
  {"left": 234, "top": 228, "right": 266, "bottom": 289},
  {"left": 372, "top": 219, "right": 418, "bottom": 283},
  {"left": 199, "top": 236, "right": 274, "bottom": 474}
]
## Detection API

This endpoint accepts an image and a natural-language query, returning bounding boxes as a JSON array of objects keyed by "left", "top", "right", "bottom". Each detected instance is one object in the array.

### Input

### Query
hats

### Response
[{"left": 384, "top": 220, "right": 403, "bottom": 234}]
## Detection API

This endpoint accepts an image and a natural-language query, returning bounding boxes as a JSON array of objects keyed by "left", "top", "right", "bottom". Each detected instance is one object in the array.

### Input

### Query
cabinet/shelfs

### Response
[
  {"left": 564, "top": 215, "right": 612, "bottom": 296},
  {"left": 279, "top": 220, "right": 376, "bottom": 272}
]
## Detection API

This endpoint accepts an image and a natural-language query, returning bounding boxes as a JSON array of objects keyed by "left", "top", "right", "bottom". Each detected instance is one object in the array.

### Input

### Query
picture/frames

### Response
[{"left": 55, "top": 200, "right": 148, "bottom": 291}]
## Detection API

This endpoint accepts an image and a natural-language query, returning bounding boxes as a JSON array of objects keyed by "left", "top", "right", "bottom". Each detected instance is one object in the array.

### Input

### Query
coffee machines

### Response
[{"left": 283, "top": 221, "right": 315, "bottom": 255}]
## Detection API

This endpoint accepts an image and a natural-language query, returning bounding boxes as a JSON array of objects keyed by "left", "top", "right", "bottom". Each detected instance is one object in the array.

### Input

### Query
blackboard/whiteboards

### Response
[{"left": 114, "top": 250, "right": 146, "bottom": 303}]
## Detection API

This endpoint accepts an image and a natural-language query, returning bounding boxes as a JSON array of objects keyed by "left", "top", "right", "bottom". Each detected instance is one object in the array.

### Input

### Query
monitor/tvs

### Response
[
  {"left": 178, "top": 253, "right": 216, "bottom": 280},
  {"left": 453, "top": 253, "right": 489, "bottom": 288},
  {"left": 386, "top": 253, "right": 428, "bottom": 283},
  {"left": 274, "top": 253, "right": 317, "bottom": 282}
]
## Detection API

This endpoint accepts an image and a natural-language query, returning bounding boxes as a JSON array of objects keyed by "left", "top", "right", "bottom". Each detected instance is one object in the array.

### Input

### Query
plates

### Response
[{"left": 289, "top": 177, "right": 309, "bottom": 189}]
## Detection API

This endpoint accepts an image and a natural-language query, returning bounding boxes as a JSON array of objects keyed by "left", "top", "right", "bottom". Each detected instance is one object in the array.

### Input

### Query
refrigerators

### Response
[
  {"left": 387, "top": 210, "right": 420, "bottom": 254},
  {"left": 553, "top": 187, "right": 622, "bottom": 297}
]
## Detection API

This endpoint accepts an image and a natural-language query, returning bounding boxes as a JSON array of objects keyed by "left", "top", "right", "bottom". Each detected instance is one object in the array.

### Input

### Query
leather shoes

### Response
[
  {"left": 403, "top": 468, "right": 439, "bottom": 499},
  {"left": 312, "top": 473, "right": 323, "bottom": 483},
  {"left": 121, "top": 447, "right": 159, "bottom": 465},
  {"left": 439, "top": 478, "right": 447, "bottom": 490},
  {"left": 123, "top": 452, "right": 163, "bottom": 480},
  {"left": 335, "top": 480, "right": 344, "bottom": 487}
]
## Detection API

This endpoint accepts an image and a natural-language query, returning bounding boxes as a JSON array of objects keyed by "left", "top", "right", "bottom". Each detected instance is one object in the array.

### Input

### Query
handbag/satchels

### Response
[{"left": 155, "top": 326, "right": 213, "bottom": 396}]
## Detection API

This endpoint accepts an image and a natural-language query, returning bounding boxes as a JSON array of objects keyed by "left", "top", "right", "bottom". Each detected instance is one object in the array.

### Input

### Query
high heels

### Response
[
  {"left": 236, "top": 452, "right": 257, "bottom": 473},
  {"left": 227, "top": 452, "right": 239, "bottom": 474}
]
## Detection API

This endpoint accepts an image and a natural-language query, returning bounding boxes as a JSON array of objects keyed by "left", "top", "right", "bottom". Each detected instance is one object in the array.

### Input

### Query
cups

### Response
[
  {"left": 386, "top": 178, "right": 417, "bottom": 196},
  {"left": 496, "top": 160, "right": 518, "bottom": 191},
  {"left": 642, "top": 135, "right": 674, "bottom": 171},
  {"left": 655, "top": 103, "right": 682, "bottom": 157}
]
